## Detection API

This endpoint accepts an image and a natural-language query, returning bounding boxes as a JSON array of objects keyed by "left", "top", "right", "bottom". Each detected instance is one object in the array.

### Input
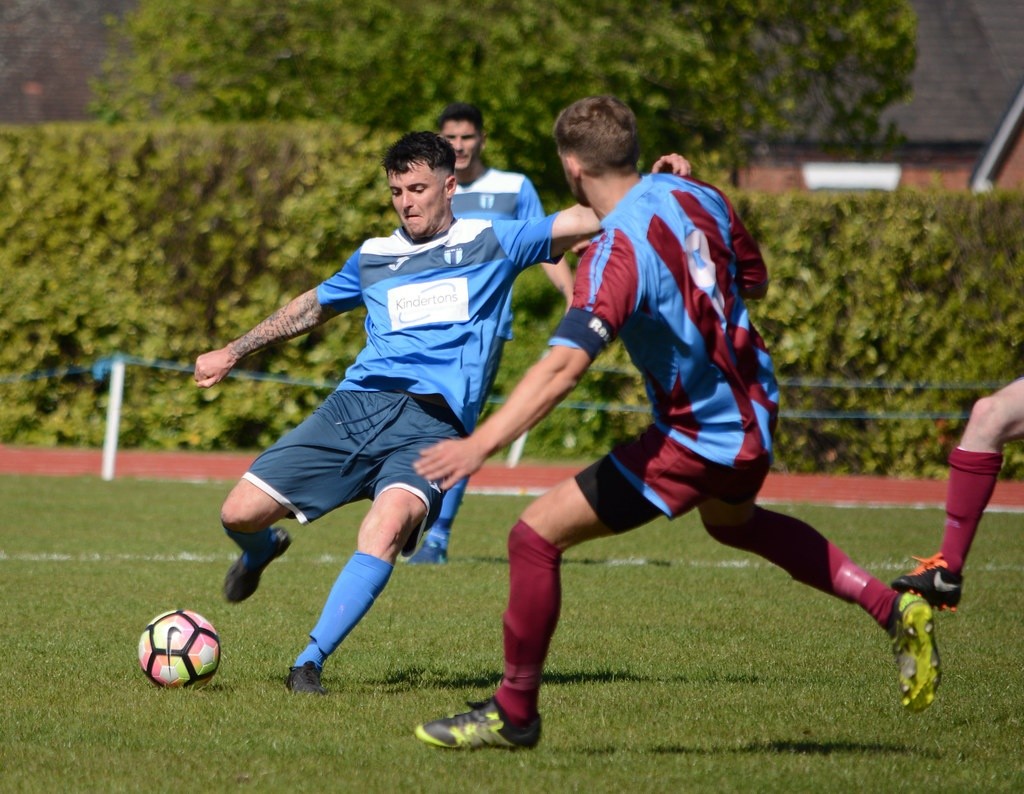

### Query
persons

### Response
[
  {"left": 408, "top": 101, "right": 576, "bottom": 562},
  {"left": 194, "top": 131, "right": 693, "bottom": 694},
  {"left": 413, "top": 95, "right": 940, "bottom": 749},
  {"left": 890, "top": 377, "right": 1024, "bottom": 612}
]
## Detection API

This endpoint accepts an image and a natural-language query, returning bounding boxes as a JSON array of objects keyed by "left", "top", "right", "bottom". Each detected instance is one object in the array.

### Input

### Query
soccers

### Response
[{"left": 137, "top": 609, "right": 222, "bottom": 690}]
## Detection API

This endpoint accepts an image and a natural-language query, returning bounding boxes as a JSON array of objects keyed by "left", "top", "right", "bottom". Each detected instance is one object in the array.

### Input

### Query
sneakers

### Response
[
  {"left": 890, "top": 552, "right": 965, "bottom": 611},
  {"left": 286, "top": 661, "right": 325, "bottom": 694},
  {"left": 415, "top": 695, "right": 541, "bottom": 750},
  {"left": 884, "top": 592, "right": 942, "bottom": 713},
  {"left": 404, "top": 541, "right": 447, "bottom": 566},
  {"left": 224, "top": 526, "right": 292, "bottom": 602}
]
